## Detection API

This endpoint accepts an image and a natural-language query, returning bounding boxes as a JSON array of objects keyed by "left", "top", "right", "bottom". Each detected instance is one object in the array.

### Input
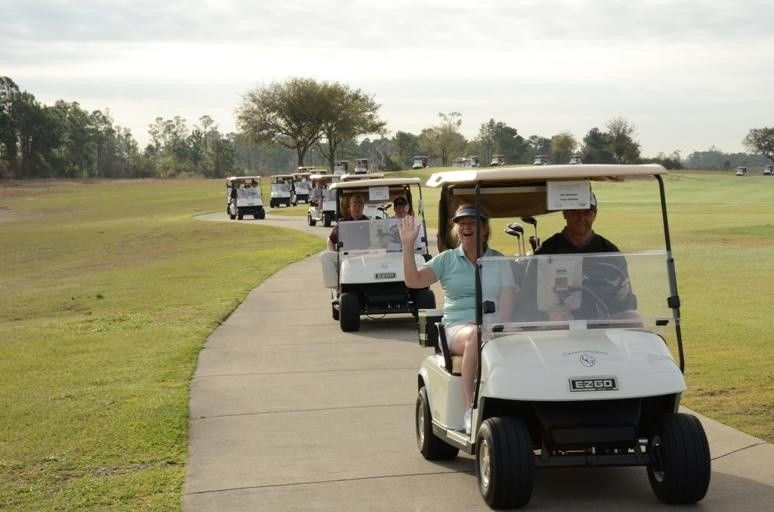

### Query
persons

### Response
[
  {"left": 311, "top": 180, "right": 327, "bottom": 209},
  {"left": 518, "top": 190, "right": 642, "bottom": 328},
  {"left": 399, "top": 203, "right": 516, "bottom": 434},
  {"left": 231, "top": 180, "right": 259, "bottom": 210},
  {"left": 327, "top": 194, "right": 427, "bottom": 255}
]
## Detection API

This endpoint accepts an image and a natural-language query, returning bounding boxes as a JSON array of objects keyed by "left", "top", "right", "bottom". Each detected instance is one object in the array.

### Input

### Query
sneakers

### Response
[
  {"left": 464, "top": 408, "right": 472, "bottom": 435},
  {"left": 332, "top": 297, "right": 339, "bottom": 304}
]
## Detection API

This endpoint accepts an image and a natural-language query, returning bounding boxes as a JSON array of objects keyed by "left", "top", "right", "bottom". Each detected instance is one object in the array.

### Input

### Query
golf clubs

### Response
[
  {"left": 504, "top": 217, "right": 540, "bottom": 256},
  {"left": 376, "top": 204, "right": 392, "bottom": 220}
]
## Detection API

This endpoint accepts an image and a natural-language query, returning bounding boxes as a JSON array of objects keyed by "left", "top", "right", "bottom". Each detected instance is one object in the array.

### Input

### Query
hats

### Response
[
  {"left": 394, "top": 197, "right": 408, "bottom": 208},
  {"left": 591, "top": 192, "right": 597, "bottom": 213},
  {"left": 452, "top": 208, "right": 488, "bottom": 223},
  {"left": 350, "top": 194, "right": 364, "bottom": 204}
]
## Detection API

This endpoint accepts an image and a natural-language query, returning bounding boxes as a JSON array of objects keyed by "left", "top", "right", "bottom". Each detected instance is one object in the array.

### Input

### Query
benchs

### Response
[{"left": 433, "top": 322, "right": 463, "bottom": 377}]
